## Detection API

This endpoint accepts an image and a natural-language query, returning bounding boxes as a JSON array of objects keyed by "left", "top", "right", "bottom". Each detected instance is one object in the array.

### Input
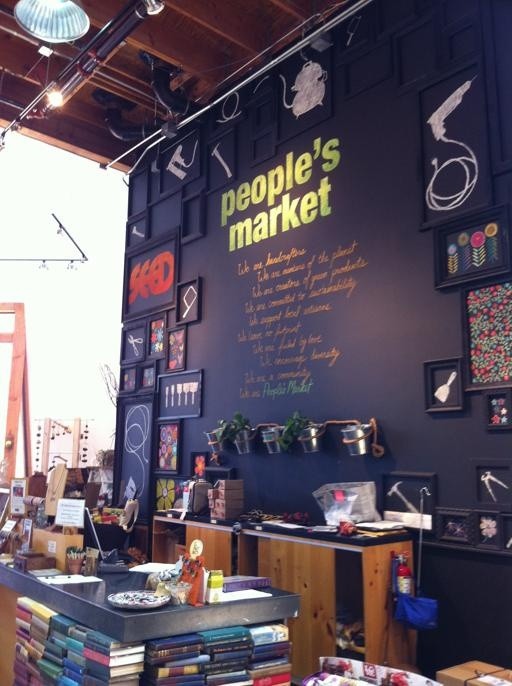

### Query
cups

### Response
[
  {"left": 67, "top": 559, "right": 83, "bottom": 574},
  {"left": 87, "top": 470, "right": 101, "bottom": 483},
  {"left": 165, "top": 583, "right": 193, "bottom": 608}
]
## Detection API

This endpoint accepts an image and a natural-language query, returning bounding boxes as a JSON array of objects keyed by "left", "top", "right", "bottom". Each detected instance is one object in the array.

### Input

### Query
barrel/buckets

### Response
[
  {"left": 340, "top": 423, "right": 372, "bottom": 456},
  {"left": 234, "top": 427, "right": 257, "bottom": 455},
  {"left": 298, "top": 423, "right": 321, "bottom": 454},
  {"left": 260, "top": 424, "right": 284, "bottom": 455},
  {"left": 203, "top": 430, "right": 223, "bottom": 453}
]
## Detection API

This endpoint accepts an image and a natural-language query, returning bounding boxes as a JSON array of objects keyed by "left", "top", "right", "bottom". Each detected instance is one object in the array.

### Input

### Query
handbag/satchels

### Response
[{"left": 392, "top": 558, "right": 439, "bottom": 631}]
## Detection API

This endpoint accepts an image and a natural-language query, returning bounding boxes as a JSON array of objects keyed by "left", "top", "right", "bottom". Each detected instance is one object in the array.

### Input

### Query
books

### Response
[
  {"left": 14, "top": 597, "right": 292, "bottom": 686},
  {"left": 222, "top": 575, "right": 272, "bottom": 592}
]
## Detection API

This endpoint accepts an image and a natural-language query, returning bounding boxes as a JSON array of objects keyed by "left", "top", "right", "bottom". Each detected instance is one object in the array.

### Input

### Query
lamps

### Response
[
  {"left": 36, "top": 46, "right": 58, "bottom": 59},
  {"left": 14, "top": 0, "right": 90, "bottom": 45}
]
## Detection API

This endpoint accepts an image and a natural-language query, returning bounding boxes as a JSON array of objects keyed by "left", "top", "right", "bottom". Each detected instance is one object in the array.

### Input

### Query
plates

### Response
[{"left": 107, "top": 590, "right": 171, "bottom": 610}]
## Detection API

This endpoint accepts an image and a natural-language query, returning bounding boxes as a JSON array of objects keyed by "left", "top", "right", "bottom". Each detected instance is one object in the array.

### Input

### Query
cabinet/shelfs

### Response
[{"left": 152, "top": 517, "right": 419, "bottom": 686}]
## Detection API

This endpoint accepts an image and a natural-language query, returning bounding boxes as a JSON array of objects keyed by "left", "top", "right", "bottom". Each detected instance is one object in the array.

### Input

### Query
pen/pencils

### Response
[{"left": 66, "top": 546, "right": 85, "bottom": 560}]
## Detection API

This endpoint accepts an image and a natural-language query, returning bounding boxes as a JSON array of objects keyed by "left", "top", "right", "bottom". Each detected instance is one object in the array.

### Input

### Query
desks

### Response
[{"left": 1, "top": 564, "right": 301, "bottom": 686}]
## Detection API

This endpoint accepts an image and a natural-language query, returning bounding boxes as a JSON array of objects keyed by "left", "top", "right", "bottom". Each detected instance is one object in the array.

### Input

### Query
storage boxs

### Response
[{"left": 436, "top": 659, "right": 512, "bottom": 686}]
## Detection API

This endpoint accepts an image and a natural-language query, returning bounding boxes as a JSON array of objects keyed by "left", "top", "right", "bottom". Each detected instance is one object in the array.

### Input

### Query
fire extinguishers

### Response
[{"left": 394, "top": 552, "right": 411, "bottom": 594}]
